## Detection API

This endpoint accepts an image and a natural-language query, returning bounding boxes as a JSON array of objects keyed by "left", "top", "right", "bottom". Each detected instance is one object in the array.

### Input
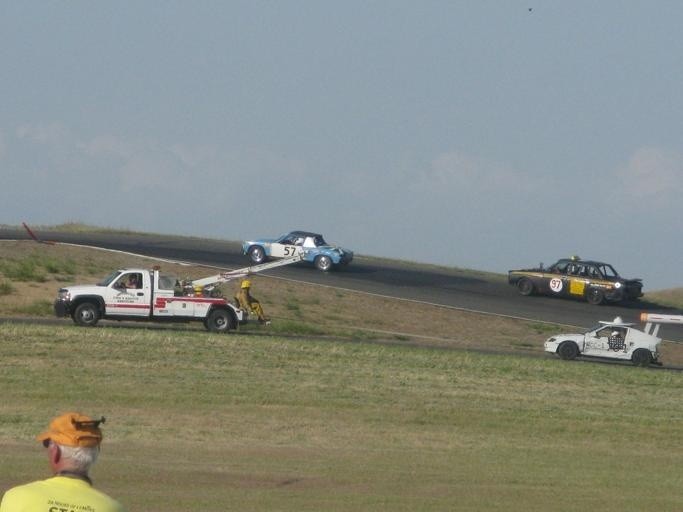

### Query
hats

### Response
[{"left": 38, "top": 413, "right": 103, "bottom": 448}]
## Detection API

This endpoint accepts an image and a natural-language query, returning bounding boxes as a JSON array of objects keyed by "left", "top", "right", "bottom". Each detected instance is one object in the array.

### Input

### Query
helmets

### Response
[{"left": 241, "top": 280, "right": 251, "bottom": 288}]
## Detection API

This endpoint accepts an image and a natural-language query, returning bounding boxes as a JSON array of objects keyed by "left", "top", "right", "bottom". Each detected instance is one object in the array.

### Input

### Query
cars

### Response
[
  {"left": 510, "top": 258, "right": 646, "bottom": 304},
  {"left": 242, "top": 229, "right": 353, "bottom": 271}
]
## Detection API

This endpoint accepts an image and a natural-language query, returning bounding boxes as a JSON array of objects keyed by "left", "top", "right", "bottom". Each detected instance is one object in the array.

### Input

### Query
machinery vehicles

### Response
[
  {"left": 544, "top": 312, "right": 683, "bottom": 366},
  {"left": 54, "top": 254, "right": 303, "bottom": 334}
]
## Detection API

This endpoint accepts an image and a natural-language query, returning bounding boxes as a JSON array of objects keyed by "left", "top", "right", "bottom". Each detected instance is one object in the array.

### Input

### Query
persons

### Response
[
  {"left": 1, "top": 410, "right": 123, "bottom": 512},
  {"left": 125, "top": 274, "right": 140, "bottom": 289},
  {"left": 237, "top": 280, "right": 271, "bottom": 323}
]
